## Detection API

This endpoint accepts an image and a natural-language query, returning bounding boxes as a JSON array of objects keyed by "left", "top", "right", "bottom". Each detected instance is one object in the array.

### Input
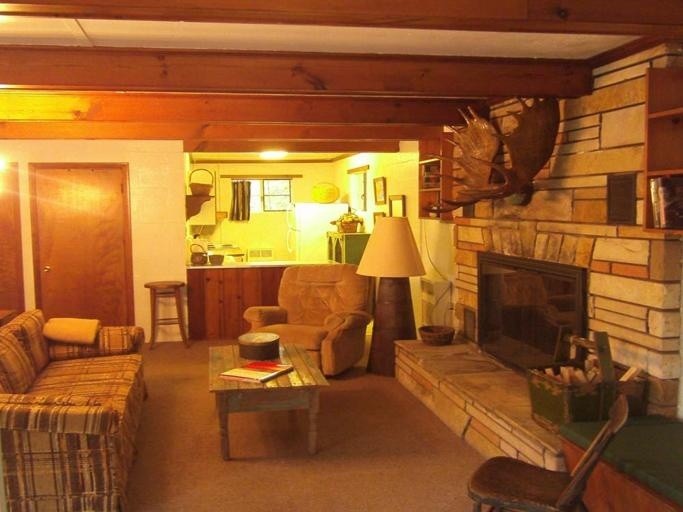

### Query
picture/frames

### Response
[
  {"left": 373, "top": 212, "right": 384, "bottom": 226},
  {"left": 373, "top": 177, "right": 387, "bottom": 206},
  {"left": 386, "top": 193, "right": 405, "bottom": 217}
]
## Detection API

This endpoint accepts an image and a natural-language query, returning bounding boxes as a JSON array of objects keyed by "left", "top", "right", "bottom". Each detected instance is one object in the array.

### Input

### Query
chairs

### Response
[
  {"left": 466, "top": 394, "right": 628, "bottom": 508},
  {"left": 241, "top": 264, "right": 373, "bottom": 378}
]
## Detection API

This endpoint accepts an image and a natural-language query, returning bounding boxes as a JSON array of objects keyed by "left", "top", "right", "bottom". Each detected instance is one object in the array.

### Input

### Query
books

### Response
[
  {"left": 650, "top": 177, "right": 662, "bottom": 228},
  {"left": 658, "top": 176, "right": 683, "bottom": 229},
  {"left": 219, "top": 358, "right": 293, "bottom": 382}
]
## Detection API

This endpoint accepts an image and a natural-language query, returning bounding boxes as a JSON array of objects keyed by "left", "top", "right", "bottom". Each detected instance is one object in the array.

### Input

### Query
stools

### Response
[{"left": 143, "top": 280, "right": 191, "bottom": 351}]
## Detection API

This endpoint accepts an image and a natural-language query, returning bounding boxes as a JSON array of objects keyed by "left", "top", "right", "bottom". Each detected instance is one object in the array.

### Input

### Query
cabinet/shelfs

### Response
[
  {"left": 642, "top": 65, "right": 682, "bottom": 235},
  {"left": 414, "top": 133, "right": 453, "bottom": 223}
]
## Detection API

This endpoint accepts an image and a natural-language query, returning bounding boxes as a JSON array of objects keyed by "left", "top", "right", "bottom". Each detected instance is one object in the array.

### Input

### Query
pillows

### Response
[{"left": 40, "top": 313, "right": 101, "bottom": 345}]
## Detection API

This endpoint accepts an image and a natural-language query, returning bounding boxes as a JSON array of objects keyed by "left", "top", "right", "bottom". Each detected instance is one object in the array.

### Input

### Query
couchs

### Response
[{"left": 1, "top": 305, "right": 145, "bottom": 511}]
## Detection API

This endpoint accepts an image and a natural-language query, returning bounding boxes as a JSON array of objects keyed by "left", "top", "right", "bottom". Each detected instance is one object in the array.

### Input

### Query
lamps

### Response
[{"left": 355, "top": 218, "right": 426, "bottom": 378}]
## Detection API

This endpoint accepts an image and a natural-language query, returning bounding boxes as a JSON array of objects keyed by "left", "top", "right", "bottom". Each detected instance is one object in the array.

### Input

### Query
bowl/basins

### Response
[
  {"left": 418, "top": 325, "right": 455, "bottom": 345},
  {"left": 208, "top": 255, "right": 224, "bottom": 266}
]
